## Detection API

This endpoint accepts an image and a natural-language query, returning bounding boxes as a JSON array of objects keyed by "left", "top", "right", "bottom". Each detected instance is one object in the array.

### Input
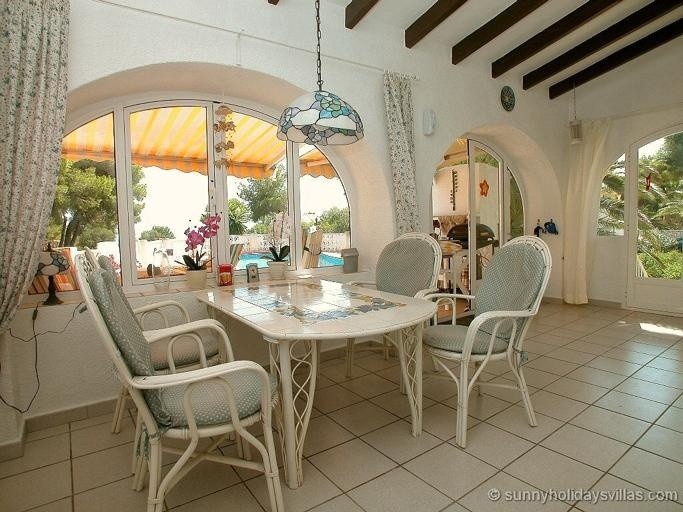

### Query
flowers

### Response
[{"left": 173, "top": 211, "right": 227, "bottom": 269}]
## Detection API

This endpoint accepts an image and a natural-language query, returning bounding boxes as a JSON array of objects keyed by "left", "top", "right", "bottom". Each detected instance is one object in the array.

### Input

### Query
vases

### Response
[{"left": 184, "top": 268, "right": 206, "bottom": 290}]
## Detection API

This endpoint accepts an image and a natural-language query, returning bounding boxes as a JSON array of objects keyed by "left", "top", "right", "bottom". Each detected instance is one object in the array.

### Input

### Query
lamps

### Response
[
  {"left": 569, "top": 83, "right": 584, "bottom": 145},
  {"left": 33, "top": 239, "right": 69, "bottom": 305},
  {"left": 276, "top": 2, "right": 364, "bottom": 149}
]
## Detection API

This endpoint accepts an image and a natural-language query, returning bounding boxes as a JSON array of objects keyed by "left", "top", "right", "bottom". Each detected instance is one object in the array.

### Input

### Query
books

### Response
[{"left": 26, "top": 246, "right": 81, "bottom": 295}]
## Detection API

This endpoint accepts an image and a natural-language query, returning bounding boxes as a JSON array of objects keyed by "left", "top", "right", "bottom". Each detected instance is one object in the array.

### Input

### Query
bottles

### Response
[{"left": 151, "top": 250, "right": 171, "bottom": 293}]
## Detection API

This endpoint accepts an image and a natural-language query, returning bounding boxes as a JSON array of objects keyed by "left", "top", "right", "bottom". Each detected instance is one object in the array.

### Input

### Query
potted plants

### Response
[{"left": 256, "top": 242, "right": 292, "bottom": 279}]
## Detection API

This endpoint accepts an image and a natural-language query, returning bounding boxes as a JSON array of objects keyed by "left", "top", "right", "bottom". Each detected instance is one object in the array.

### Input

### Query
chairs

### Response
[
  {"left": 416, "top": 233, "right": 554, "bottom": 452},
  {"left": 341, "top": 229, "right": 448, "bottom": 396},
  {"left": 80, "top": 241, "right": 251, "bottom": 493},
  {"left": 68, "top": 254, "right": 286, "bottom": 510}
]
